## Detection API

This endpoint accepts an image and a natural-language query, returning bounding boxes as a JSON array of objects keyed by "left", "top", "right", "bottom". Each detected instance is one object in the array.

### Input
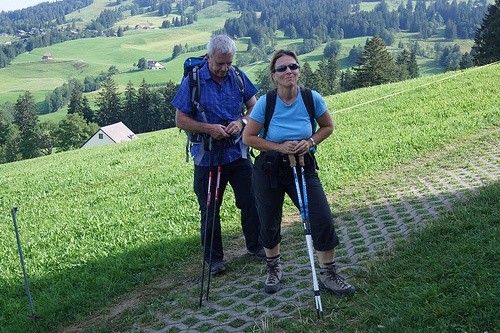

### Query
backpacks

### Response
[{"left": 183, "top": 53, "right": 245, "bottom": 144}]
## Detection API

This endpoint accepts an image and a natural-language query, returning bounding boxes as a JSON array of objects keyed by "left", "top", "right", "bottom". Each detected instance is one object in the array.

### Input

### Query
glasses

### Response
[{"left": 274, "top": 63, "right": 300, "bottom": 73}]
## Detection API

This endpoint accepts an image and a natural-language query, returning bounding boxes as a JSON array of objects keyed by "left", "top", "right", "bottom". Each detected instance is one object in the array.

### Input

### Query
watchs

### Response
[
  {"left": 310, "top": 138, "right": 316, "bottom": 146},
  {"left": 240, "top": 116, "right": 249, "bottom": 126}
]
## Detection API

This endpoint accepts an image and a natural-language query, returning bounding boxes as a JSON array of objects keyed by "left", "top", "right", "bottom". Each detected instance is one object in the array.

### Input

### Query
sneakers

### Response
[
  {"left": 206, "top": 262, "right": 225, "bottom": 275},
  {"left": 264, "top": 254, "right": 285, "bottom": 294},
  {"left": 246, "top": 249, "right": 266, "bottom": 260},
  {"left": 317, "top": 263, "right": 356, "bottom": 297}
]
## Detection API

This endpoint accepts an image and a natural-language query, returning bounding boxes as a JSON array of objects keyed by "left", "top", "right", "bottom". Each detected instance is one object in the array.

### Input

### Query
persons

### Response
[
  {"left": 242, "top": 48, "right": 355, "bottom": 297},
  {"left": 171, "top": 35, "right": 266, "bottom": 273}
]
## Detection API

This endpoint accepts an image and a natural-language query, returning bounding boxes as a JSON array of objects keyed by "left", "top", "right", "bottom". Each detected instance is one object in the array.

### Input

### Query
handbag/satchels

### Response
[{"left": 202, "top": 118, "right": 234, "bottom": 151}]
